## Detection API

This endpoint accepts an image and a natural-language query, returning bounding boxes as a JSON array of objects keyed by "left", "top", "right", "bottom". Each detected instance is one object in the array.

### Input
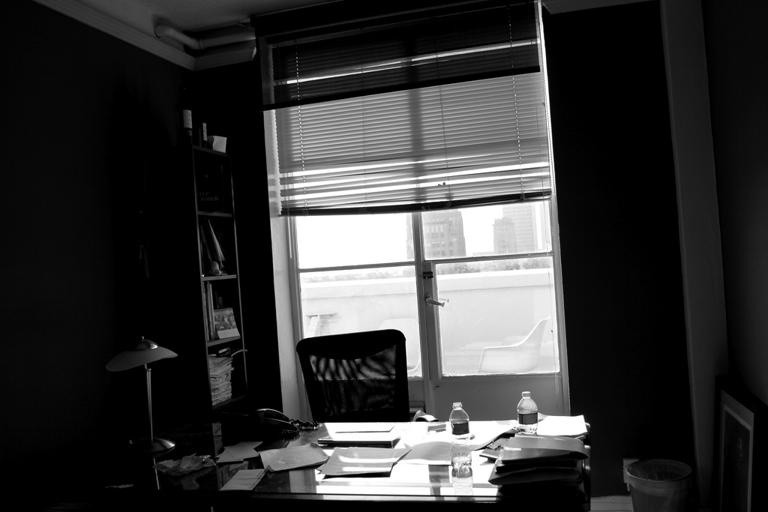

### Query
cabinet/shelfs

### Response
[{"left": 170, "top": 142, "right": 253, "bottom": 447}]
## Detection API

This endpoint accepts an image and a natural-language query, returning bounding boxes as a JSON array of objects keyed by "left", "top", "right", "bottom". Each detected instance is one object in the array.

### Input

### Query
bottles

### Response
[
  {"left": 450, "top": 401, "right": 471, "bottom": 466},
  {"left": 516, "top": 391, "right": 539, "bottom": 435}
]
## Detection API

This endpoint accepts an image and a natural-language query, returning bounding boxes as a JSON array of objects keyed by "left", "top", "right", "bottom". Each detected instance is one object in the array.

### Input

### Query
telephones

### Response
[{"left": 241, "top": 408, "right": 297, "bottom": 441}]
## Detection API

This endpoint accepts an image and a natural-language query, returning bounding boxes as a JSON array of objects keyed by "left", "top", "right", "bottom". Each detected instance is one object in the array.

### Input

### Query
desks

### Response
[{"left": 84, "top": 413, "right": 593, "bottom": 510}]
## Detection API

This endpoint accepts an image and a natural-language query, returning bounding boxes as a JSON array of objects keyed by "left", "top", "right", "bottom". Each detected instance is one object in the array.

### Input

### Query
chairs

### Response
[{"left": 295, "top": 329, "right": 412, "bottom": 421}]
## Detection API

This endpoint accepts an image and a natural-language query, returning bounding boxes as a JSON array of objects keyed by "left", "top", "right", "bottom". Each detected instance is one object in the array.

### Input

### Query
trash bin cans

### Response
[{"left": 625, "top": 458, "right": 692, "bottom": 512}]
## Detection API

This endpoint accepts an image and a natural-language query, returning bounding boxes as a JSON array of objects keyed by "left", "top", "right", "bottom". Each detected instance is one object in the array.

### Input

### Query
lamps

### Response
[{"left": 104, "top": 333, "right": 180, "bottom": 457}]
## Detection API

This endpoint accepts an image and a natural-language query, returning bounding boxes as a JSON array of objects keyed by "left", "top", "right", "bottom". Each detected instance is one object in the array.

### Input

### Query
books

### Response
[
  {"left": 198, "top": 216, "right": 243, "bottom": 408},
  {"left": 488, "top": 431, "right": 589, "bottom": 505}
]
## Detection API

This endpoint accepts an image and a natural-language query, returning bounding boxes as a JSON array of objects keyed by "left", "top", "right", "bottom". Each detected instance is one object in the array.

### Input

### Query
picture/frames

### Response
[{"left": 714, "top": 366, "right": 767, "bottom": 512}]
